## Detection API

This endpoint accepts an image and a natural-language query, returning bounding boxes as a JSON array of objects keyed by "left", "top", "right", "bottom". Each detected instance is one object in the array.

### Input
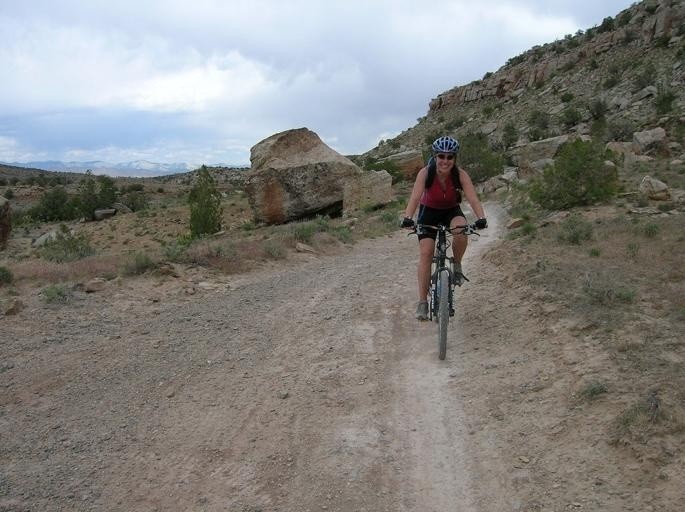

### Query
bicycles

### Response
[{"left": 404, "top": 220, "right": 489, "bottom": 361}]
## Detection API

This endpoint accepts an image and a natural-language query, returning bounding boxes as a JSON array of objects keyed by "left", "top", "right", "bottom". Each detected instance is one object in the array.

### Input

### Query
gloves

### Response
[
  {"left": 474, "top": 218, "right": 487, "bottom": 229},
  {"left": 401, "top": 215, "right": 415, "bottom": 227}
]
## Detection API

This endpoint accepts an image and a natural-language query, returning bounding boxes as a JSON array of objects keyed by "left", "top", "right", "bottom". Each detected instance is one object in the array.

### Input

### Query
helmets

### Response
[{"left": 432, "top": 135, "right": 460, "bottom": 154}]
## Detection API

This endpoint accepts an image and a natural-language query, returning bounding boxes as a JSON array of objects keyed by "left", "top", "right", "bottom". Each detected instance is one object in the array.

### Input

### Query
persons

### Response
[{"left": 400, "top": 136, "right": 488, "bottom": 320}]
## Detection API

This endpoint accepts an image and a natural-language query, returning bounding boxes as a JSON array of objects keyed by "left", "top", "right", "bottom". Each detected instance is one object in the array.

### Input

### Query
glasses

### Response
[{"left": 435, "top": 154, "right": 456, "bottom": 160}]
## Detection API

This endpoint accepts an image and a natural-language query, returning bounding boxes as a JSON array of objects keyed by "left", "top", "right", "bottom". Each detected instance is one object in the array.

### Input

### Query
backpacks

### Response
[{"left": 425, "top": 163, "right": 464, "bottom": 204}]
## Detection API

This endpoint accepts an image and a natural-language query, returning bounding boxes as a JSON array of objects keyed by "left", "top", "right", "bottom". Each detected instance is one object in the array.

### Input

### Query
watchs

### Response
[{"left": 405, "top": 215, "right": 412, "bottom": 221}]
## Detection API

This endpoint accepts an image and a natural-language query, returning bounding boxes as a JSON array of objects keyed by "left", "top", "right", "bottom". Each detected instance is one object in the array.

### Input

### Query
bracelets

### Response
[{"left": 480, "top": 217, "right": 485, "bottom": 219}]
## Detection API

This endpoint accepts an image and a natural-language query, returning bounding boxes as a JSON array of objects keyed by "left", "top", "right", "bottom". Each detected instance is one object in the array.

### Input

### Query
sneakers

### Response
[
  {"left": 415, "top": 301, "right": 429, "bottom": 320},
  {"left": 451, "top": 263, "right": 464, "bottom": 286}
]
